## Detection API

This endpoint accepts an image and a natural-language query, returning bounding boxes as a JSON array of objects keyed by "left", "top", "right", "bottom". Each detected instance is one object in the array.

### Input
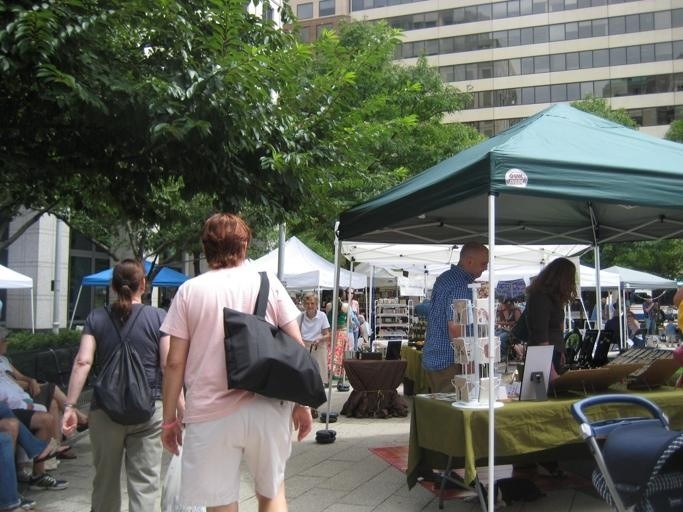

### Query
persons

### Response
[
  {"left": 511, "top": 258, "right": 576, "bottom": 479},
  {"left": 159, "top": 213, "right": 314, "bottom": 510},
  {"left": 495, "top": 299, "right": 523, "bottom": 360},
  {"left": 295, "top": 287, "right": 372, "bottom": 418},
  {"left": 61, "top": 258, "right": 184, "bottom": 512},
  {"left": 590, "top": 285, "right": 683, "bottom": 363},
  {"left": 420, "top": 242, "right": 488, "bottom": 489},
  {"left": 0, "top": 326, "right": 89, "bottom": 511},
  {"left": 415, "top": 289, "right": 433, "bottom": 318}
]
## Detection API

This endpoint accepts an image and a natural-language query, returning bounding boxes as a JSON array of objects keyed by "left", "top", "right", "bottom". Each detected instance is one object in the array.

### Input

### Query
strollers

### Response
[{"left": 569, "top": 392, "right": 683, "bottom": 511}]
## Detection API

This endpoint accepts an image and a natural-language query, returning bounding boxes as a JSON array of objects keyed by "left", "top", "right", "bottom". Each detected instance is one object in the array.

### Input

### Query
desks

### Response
[
  {"left": 341, "top": 360, "right": 409, "bottom": 420},
  {"left": 400, "top": 345, "right": 491, "bottom": 395},
  {"left": 407, "top": 384, "right": 683, "bottom": 511}
]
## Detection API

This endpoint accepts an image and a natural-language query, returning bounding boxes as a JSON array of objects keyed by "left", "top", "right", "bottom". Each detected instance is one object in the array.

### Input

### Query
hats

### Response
[{"left": 0, "top": 325, "right": 11, "bottom": 341}]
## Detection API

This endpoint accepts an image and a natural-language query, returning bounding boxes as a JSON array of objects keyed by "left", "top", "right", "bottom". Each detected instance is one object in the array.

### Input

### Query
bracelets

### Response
[{"left": 157, "top": 417, "right": 179, "bottom": 429}]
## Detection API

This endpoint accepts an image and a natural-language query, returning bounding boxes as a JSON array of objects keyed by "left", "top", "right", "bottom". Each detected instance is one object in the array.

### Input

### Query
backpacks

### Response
[{"left": 91, "top": 337, "right": 158, "bottom": 426}]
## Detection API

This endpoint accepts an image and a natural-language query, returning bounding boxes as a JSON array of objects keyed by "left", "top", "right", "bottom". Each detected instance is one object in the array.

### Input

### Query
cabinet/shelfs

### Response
[{"left": 374, "top": 297, "right": 413, "bottom": 343}]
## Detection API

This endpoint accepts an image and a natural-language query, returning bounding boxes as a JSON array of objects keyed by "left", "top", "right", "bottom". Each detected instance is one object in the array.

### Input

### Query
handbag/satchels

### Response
[{"left": 219, "top": 304, "right": 329, "bottom": 412}]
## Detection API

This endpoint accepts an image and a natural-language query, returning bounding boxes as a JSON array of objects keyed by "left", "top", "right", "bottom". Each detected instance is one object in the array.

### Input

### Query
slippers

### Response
[{"left": 32, "top": 442, "right": 72, "bottom": 466}]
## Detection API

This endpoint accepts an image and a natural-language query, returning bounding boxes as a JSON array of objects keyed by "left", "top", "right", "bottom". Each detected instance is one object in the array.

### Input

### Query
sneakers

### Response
[
  {"left": 19, "top": 494, "right": 37, "bottom": 509},
  {"left": 54, "top": 445, "right": 79, "bottom": 460},
  {"left": 27, "top": 471, "right": 69, "bottom": 491}
]
